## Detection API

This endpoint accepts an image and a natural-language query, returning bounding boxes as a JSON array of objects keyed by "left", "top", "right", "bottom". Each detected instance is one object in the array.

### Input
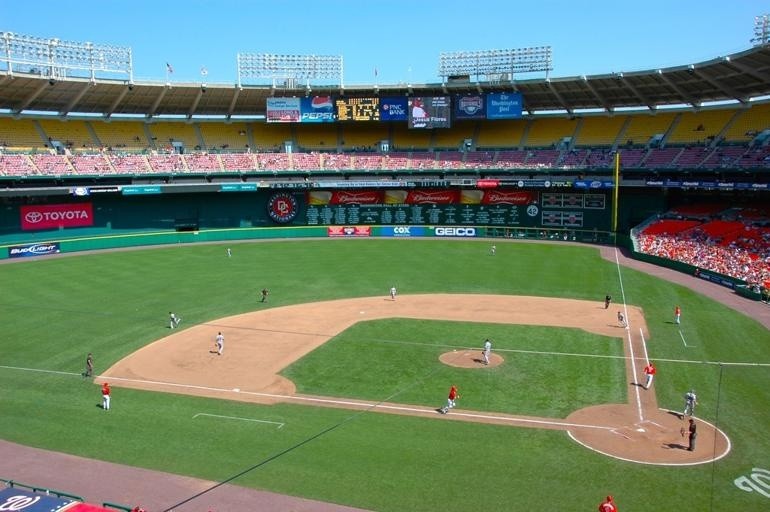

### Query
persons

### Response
[
  {"left": 643, "top": 361, "right": 657, "bottom": 389},
  {"left": 102, "top": 383, "right": 111, "bottom": 409},
  {"left": 82, "top": 352, "right": 94, "bottom": 378},
  {"left": 389, "top": 284, "right": 396, "bottom": 301},
  {"left": 215, "top": 331, "right": 225, "bottom": 355},
  {"left": 633, "top": 205, "right": 770, "bottom": 297},
  {"left": 226, "top": 247, "right": 232, "bottom": 257},
  {"left": 168, "top": 311, "right": 181, "bottom": 330},
  {"left": 481, "top": 338, "right": 491, "bottom": 365},
  {"left": 605, "top": 294, "right": 613, "bottom": 311},
  {"left": 683, "top": 388, "right": 698, "bottom": 418},
  {"left": 674, "top": 304, "right": 681, "bottom": 323},
  {"left": 260, "top": 288, "right": 270, "bottom": 303},
  {"left": 447, "top": 384, "right": 462, "bottom": 408},
  {"left": 683, "top": 419, "right": 698, "bottom": 451},
  {"left": 617, "top": 311, "right": 628, "bottom": 328},
  {"left": 598, "top": 495, "right": 618, "bottom": 512}
]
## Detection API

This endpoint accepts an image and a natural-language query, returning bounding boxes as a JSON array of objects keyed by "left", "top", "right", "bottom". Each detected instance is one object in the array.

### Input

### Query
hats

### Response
[{"left": 607, "top": 495, "right": 613, "bottom": 501}]
